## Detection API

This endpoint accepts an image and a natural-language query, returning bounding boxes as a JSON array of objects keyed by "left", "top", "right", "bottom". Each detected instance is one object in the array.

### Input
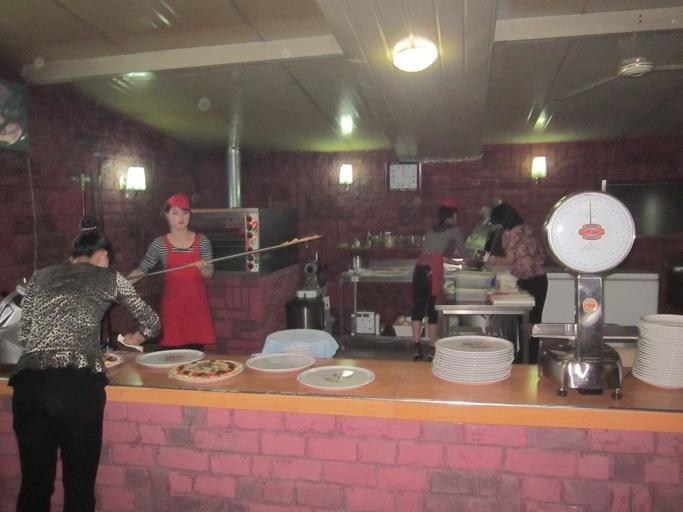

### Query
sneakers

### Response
[
  {"left": 411, "top": 346, "right": 426, "bottom": 359},
  {"left": 428, "top": 349, "right": 436, "bottom": 359},
  {"left": 514, "top": 356, "right": 523, "bottom": 364},
  {"left": 527, "top": 359, "right": 538, "bottom": 364}
]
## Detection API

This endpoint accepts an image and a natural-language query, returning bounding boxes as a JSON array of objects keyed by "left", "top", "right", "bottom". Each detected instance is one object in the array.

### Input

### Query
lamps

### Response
[
  {"left": 531, "top": 155, "right": 547, "bottom": 185},
  {"left": 120, "top": 164, "right": 147, "bottom": 198},
  {"left": 336, "top": 162, "right": 354, "bottom": 191}
]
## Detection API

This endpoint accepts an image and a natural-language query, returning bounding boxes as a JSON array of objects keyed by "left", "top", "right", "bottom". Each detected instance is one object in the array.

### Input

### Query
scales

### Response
[{"left": 527, "top": 189, "right": 640, "bottom": 399}]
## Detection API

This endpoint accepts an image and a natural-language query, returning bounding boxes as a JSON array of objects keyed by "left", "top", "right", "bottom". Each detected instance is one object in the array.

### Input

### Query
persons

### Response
[
  {"left": 126, "top": 192, "right": 219, "bottom": 353},
  {"left": 8, "top": 216, "right": 161, "bottom": 512},
  {"left": 490, "top": 203, "right": 549, "bottom": 363},
  {"left": 412, "top": 207, "right": 482, "bottom": 359}
]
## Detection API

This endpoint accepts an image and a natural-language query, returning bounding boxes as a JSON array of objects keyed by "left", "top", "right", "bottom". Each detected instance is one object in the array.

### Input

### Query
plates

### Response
[
  {"left": 168, "top": 360, "right": 244, "bottom": 382},
  {"left": 430, "top": 335, "right": 516, "bottom": 385},
  {"left": 103, "top": 352, "right": 124, "bottom": 370},
  {"left": 630, "top": 314, "right": 682, "bottom": 390},
  {"left": 245, "top": 353, "right": 314, "bottom": 372},
  {"left": 134, "top": 348, "right": 205, "bottom": 367},
  {"left": 297, "top": 367, "right": 376, "bottom": 388}
]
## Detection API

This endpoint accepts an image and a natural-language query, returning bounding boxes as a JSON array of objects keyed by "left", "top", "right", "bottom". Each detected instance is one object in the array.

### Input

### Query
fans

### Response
[{"left": 549, "top": 31, "right": 681, "bottom": 103}]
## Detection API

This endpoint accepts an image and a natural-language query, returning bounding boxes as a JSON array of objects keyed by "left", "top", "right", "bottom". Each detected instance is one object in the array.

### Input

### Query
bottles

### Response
[{"left": 366, "top": 231, "right": 426, "bottom": 250}]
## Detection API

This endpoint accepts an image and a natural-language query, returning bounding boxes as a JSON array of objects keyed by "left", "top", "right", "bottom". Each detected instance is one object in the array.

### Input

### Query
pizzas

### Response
[
  {"left": 170, "top": 360, "right": 241, "bottom": 380},
  {"left": 102, "top": 353, "right": 121, "bottom": 366}
]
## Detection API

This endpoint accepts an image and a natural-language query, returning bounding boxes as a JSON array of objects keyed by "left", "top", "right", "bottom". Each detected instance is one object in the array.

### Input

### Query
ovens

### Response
[{"left": 188, "top": 205, "right": 300, "bottom": 274}]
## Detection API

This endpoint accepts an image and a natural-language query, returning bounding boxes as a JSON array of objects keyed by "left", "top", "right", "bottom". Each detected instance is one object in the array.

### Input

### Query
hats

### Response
[{"left": 165, "top": 193, "right": 191, "bottom": 211}]
